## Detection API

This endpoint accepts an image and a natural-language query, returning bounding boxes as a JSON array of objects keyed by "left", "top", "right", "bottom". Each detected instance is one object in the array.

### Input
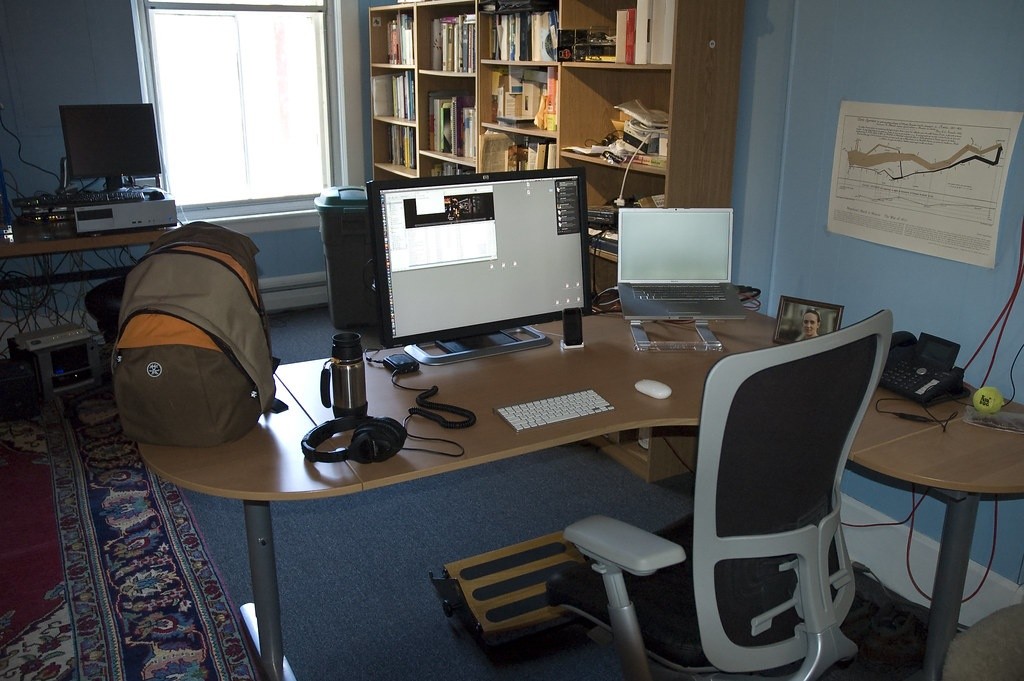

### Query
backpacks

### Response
[{"left": 110, "top": 221, "right": 290, "bottom": 447}]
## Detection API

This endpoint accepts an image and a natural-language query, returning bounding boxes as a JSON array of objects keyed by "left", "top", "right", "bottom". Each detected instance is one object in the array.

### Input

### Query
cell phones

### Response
[{"left": 562, "top": 307, "right": 583, "bottom": 346}]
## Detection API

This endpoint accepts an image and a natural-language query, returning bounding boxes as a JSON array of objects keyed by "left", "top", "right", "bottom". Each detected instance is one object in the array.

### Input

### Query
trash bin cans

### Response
[{"left": 315, "top": 184, "right": 376, "bottom": 328}]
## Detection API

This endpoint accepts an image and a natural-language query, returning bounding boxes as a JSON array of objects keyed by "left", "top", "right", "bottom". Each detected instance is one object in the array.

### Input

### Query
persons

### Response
[{"left": 801, "top": 308, "right": 820, "bottom": 341}]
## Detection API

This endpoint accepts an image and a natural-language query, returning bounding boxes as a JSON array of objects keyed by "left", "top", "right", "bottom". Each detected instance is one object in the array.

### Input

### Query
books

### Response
[
  {"left": 430, "top": 12, "right": 477, "bottom": 75},
  {"left": 390, "top": 125, "right": 419, "bottom": 169},
  {"left": 427, "top": 90, "right": 476, "bottom": 158},
  {"left": 432, "top": 160, "right": 476, "bottom": 176},
  {"left": 476, "top": 133, "right": 557, "bottom": 174},
  {"left": 613, "top": 0, "right": 675, "bottom": 65},
  {"left": 371, "top": 69, "right": 417, "bottom": 123},
  {"left": 387, "top": 8, "right": 416, "bottom": 65},
  {"left": 545, "top": 66, "right": 558, "bottom": 131},
  {"left": 493, "top": 10, "right": 559, "bottom": 61}
]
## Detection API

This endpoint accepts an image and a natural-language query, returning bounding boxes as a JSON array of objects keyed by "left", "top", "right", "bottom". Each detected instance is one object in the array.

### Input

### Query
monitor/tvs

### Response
[
  {"left": 60, "top": 103, "right": 162, "bottom": 193},
  {"left": 366, "top": 167, "right": 592, "bottom": 366}
]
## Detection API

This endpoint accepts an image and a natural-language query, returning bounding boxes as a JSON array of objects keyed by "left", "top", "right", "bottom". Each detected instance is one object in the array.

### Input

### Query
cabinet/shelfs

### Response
[{"left": 366, "top": 1, "right": 746, "bottom": 295}]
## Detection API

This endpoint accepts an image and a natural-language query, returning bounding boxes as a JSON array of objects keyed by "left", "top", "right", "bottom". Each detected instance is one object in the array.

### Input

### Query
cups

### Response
[{"left": 320, "top": 332, "right": 368, "bottom": 419}]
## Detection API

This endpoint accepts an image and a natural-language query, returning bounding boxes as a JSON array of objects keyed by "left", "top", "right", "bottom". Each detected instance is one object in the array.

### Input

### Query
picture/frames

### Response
[{"left": 775, "top": 294, "right": 844, "bottom": 345}]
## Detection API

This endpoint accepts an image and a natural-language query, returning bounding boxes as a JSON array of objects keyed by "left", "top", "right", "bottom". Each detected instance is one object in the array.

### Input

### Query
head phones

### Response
[{"left": 301, "top": 415, "right": 407, "bottom": 464}]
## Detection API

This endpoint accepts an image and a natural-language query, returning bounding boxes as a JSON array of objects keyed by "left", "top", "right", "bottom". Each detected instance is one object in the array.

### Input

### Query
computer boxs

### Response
[{"left": 6, "top": 334, "right": 103, "bottom": 400}]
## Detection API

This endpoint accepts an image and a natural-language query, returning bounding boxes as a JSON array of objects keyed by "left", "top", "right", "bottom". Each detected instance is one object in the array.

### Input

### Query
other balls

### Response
[{"left": 972, "top": 386, "right": 1003, "bottom": 413}]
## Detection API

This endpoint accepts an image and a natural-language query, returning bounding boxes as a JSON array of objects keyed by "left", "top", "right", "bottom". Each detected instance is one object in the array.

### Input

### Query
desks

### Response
[
  {"left": 135, "top": 301, "right": 1023, "bottom": 681},
  {"left": 0, "top": 220, "right": 177, "bottom": 259}
]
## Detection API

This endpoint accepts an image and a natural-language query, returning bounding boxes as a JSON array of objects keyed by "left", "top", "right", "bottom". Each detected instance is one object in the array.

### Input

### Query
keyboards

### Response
[
  {"left": 66, "top": 190, "right": 145, "bottom": 207},
  {"left": 493, "top": 390, "right": 615, "bottom": 432}
]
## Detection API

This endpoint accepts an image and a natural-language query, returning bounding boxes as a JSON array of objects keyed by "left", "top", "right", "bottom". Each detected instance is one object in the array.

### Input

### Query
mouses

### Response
[
  {"left": 148, "top": 191, "right": 165, "bottom": 200},
  {"left": 635, "top": 379, "right": 671, "bottom": 399}
]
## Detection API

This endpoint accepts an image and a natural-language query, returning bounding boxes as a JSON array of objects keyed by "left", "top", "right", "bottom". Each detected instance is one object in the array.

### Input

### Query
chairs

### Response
[{"left": 541, "top": 309, "right": 897, "bottom": 681}]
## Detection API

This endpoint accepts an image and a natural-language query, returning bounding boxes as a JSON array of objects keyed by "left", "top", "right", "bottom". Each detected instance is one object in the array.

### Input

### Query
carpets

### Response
[{"left": 0, "top": 399, "right": 262, "bottom": 681}]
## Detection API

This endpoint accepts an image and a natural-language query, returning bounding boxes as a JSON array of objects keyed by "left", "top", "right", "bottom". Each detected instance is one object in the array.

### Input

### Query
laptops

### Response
[{"left": 617, "top": 207, "right": 747, "bottom": 320}]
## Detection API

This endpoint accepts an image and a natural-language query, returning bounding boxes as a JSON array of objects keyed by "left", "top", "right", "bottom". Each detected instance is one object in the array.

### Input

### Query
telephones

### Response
[{"left": 879, "top": 330, "right": 966, "bottom": 404}]
